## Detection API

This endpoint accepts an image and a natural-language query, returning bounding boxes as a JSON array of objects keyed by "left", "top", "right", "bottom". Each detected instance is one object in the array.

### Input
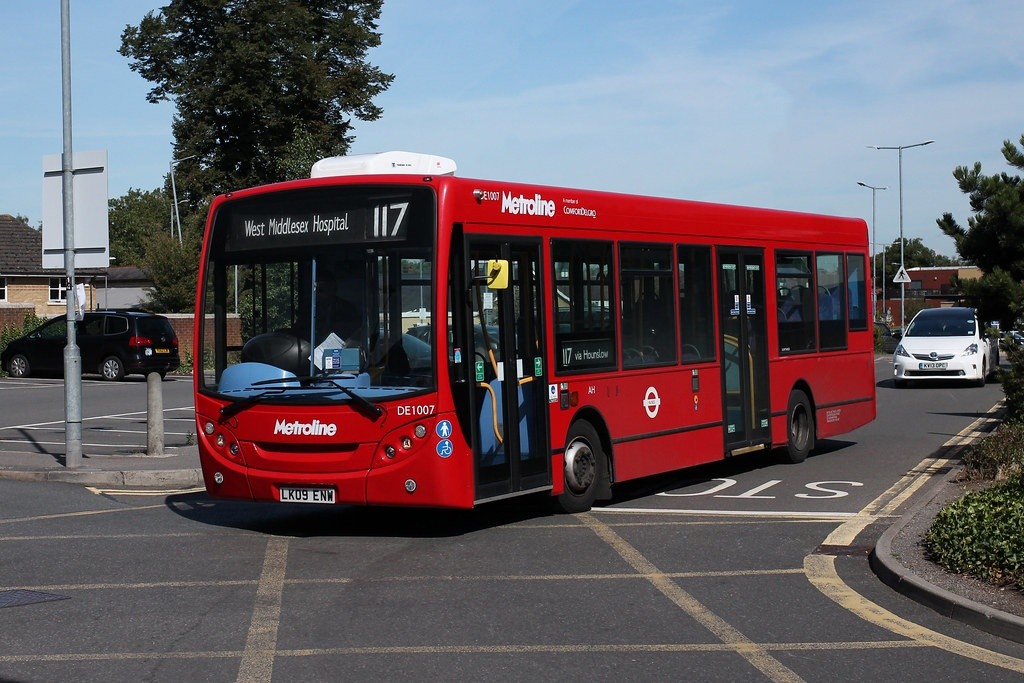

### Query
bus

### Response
[{"left": 191, "top": 152, "right": 878, "bottom": 514}]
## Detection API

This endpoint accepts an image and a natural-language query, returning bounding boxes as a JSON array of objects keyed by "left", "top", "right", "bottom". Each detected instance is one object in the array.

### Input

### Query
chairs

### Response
[
  {"left": 777, "top": 277, "right": 860, "bottom": 327},
  {"left": 558, "top": 314, "right": 703, "bottom": 376}
]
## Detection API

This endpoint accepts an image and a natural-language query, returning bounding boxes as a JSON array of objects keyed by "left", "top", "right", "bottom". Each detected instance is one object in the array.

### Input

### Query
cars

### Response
[
  {"left": 996, "top": 330, "right": 1024, "bottom": 348},
  {"left": 895, "top": 307, "right": 1000, "bottom": 388}
]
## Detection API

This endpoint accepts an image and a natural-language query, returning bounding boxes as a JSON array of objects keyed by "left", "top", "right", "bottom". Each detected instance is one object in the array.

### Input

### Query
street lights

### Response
[
  {"left": 858, "top": 181, "right": 886, "bottom": 323},
  {"left": 868, "top": 140, "right": 935, "bottom": 337},
  {"left": 170, "top": 154, "right": 198, "bottom": 247},
  {"left": 168, "top": 198, "right": 193, "bottom": 240}
]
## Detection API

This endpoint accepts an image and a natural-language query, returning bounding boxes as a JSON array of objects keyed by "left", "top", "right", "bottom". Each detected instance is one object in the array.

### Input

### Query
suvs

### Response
[{"left": 0, "top": 308, "right": 180, "bottom": 384}]
